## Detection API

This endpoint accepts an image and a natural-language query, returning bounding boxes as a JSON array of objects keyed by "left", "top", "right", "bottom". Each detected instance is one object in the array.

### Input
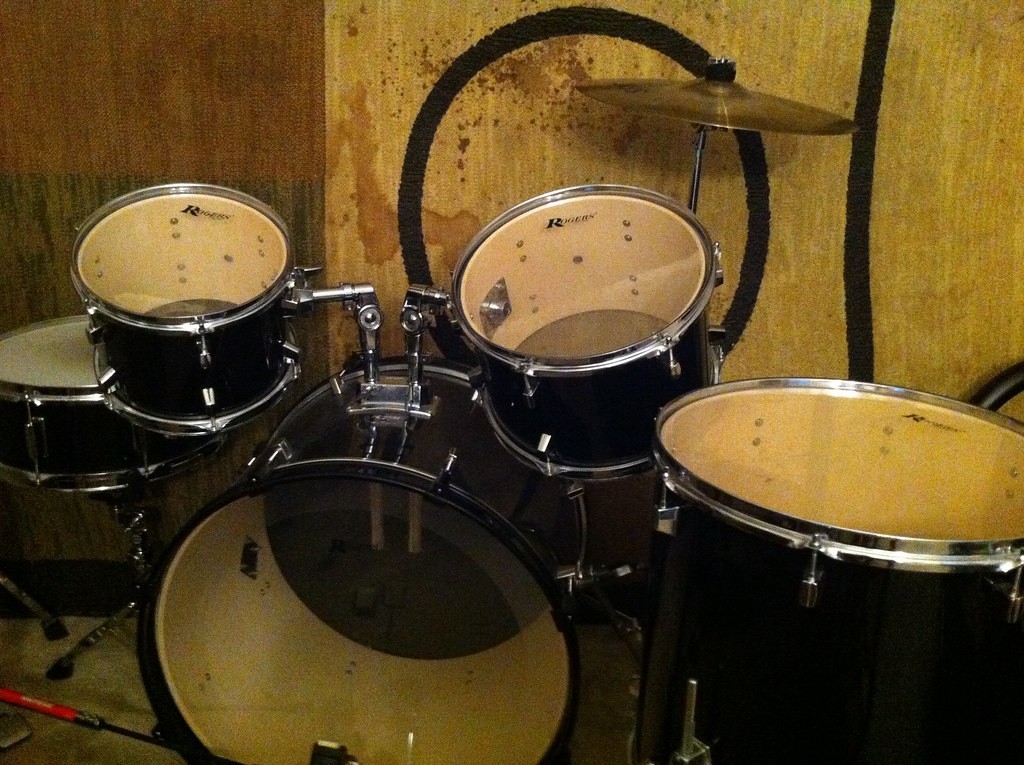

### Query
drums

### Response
[
  {"left": 136, "top": 352, "right": 590, "bottom": 765},
  {"left": 0, "top": 314, "right": 230, "bottom": 495},
  {"left": 648, "top": 376, "right": 1024, "bottom": 765},
  {"left": 452, "top": 182, "right": 720, "bottom": 477},
  {"left": 66, "top": 180, "right": 303, "bottom": 435}
]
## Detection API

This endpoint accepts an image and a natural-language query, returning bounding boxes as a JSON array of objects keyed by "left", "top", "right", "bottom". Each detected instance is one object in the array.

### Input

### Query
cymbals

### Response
[{"left": 573, "top": 73, "right": 860, "bottom": 138}]
